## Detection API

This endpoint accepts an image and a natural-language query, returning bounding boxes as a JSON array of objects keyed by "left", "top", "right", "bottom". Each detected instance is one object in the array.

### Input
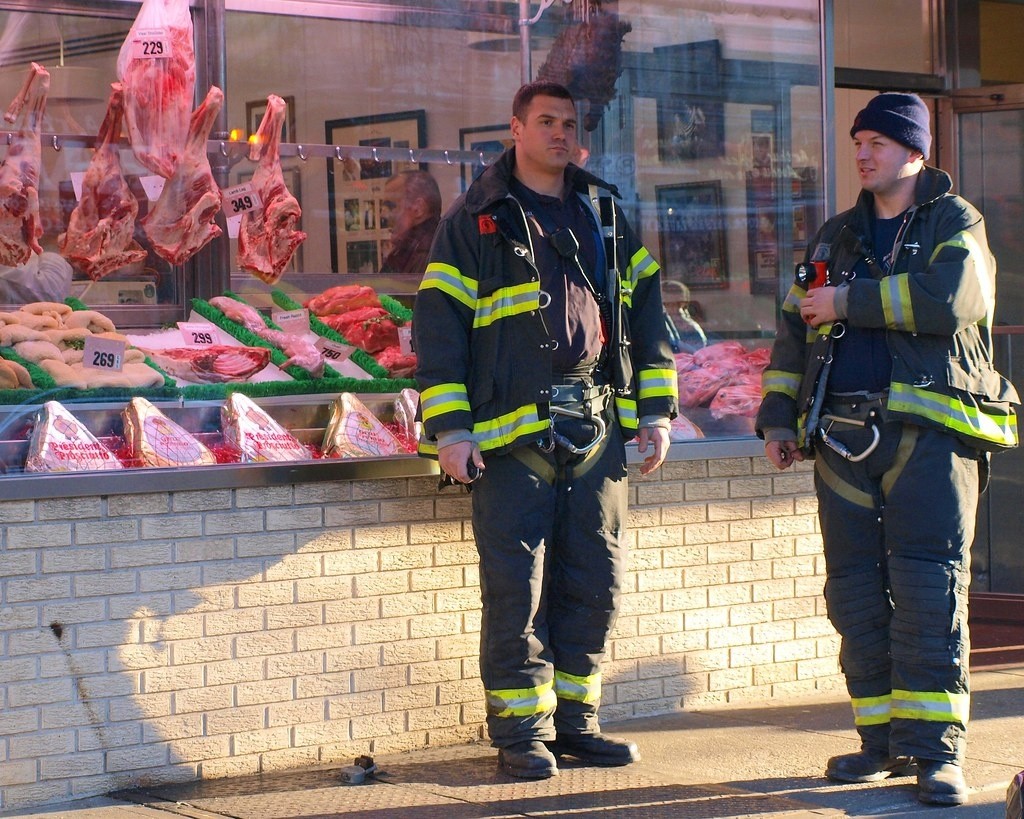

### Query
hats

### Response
[{"left": 849, "top": 92, "right": 932, "bottom": 161}]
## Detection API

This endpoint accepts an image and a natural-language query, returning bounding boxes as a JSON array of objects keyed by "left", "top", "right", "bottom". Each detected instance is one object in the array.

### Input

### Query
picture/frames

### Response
[
  {"left": 235, "top": 163, "right": 304, "bottom": 274},
  {"left": 652, "top": 40, "right": 725, "bottom": 160},
  {"left": 459, "top": 123, "right": 514, "bottom": 195},
  {"left": 324, "top": 108, "right": 430, "bottom": 275},
  {"left": 745, "top": 167, "right": 816, "bottom": 296},
  {"left": 244, "top": 94, "right": 297, "bottom": 144},
  {"left": 654, "top": 180, "right": 730, "bottom": 290}
]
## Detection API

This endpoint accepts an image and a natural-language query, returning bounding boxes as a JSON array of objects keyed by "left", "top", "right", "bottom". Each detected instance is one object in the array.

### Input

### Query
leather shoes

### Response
[
  {"left": 915, "top": 756, "right": 968, "bottom": 805},
  {"left": 825, "top": 748, "right": 918, "bottom": 784},
  {"left": 544, "top": 732, "right": 640, "bottom": 767},
  {"left": 498, "top": 740, "right": 559, "bottom": 778}
]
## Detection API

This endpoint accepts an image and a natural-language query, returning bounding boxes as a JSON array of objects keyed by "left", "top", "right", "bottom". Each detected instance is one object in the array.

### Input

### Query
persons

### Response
[
  {"left": 0, "top": 211, "right": 75, "bottom": 303},
  {"left": 753, "top": 91, "right": 996, "bottom": 804},
  {"left": 661, "top": 97, "right": 771, "bottom": 355},
  {"left": 346, "top": 201, "right": 394, "bottom": 272},
  {"left": 412, "top": 80, "right": 678, "bottom": 777},
  {"left": 379, "top": 171, "right": 444, "bottom": 272}
]
